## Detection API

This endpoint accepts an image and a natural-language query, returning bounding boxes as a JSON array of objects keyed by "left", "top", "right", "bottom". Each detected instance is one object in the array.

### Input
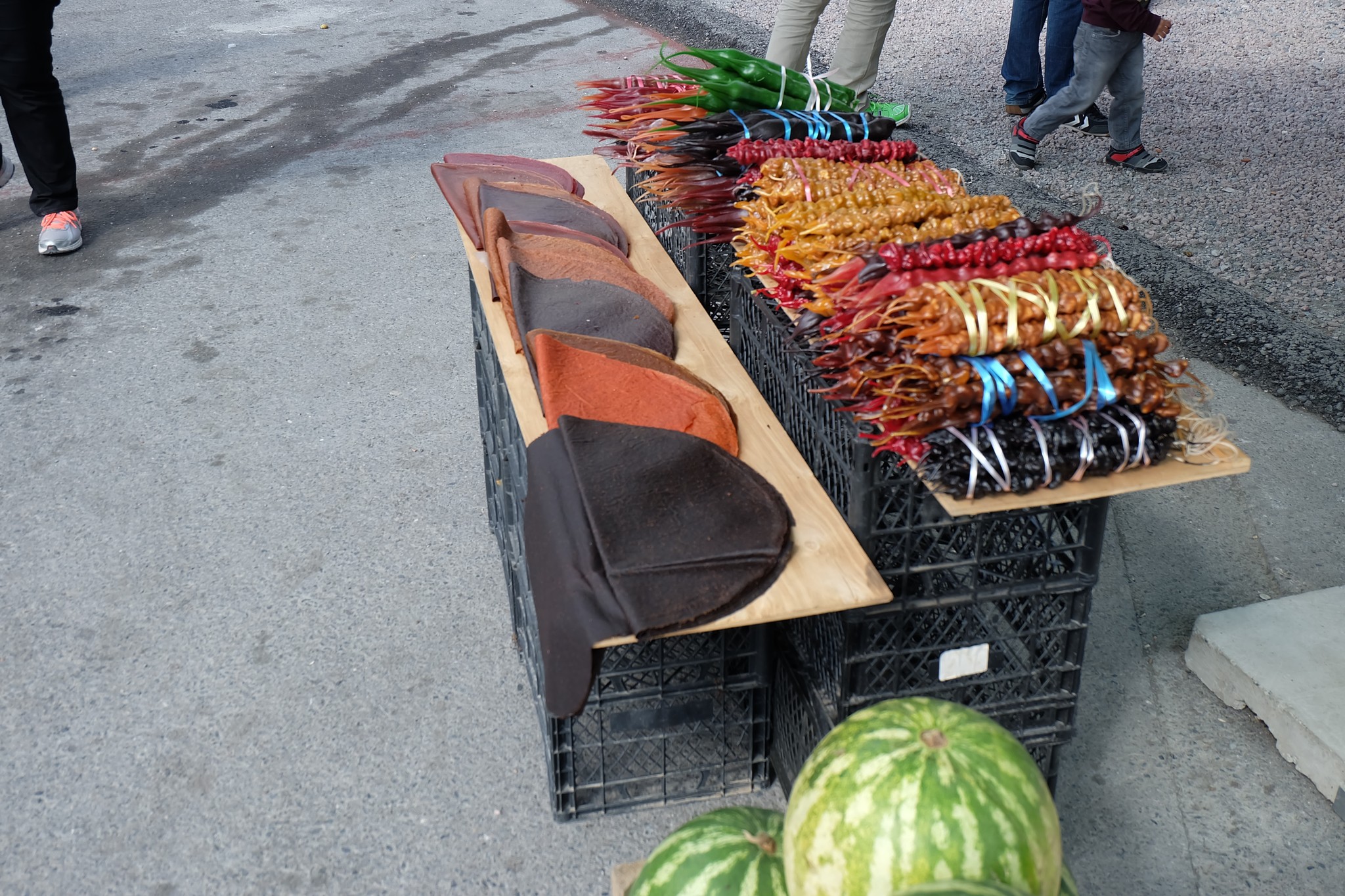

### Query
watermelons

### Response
[
  {"left": 781, "top": 698, "right": 1065, "bottom": 895},
  {"left": 863, "top": 879, "right": 1028, "bottom": 896},
  {"left": 626, "top": 807, "right": 782, "bottom": 896}
]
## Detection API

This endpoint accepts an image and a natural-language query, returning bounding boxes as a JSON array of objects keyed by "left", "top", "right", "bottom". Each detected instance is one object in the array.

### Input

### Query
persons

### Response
[
  {"left": 0, "top": 0, "right": 86, "bottom": 258},
  {"left": 1000, "top": 0, "right": 1114, "bottom": 140},
  {"left": 1008, "top": 0, "right": 1173, "bottom": 175},
  {"left": 762, "top": 0, "right": 913, "bottom": 129}
]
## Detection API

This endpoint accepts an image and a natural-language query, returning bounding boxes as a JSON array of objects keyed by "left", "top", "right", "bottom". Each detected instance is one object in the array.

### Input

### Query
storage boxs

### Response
[{"left": 473, "top": 164, "right": 1114, "bottom": 821}]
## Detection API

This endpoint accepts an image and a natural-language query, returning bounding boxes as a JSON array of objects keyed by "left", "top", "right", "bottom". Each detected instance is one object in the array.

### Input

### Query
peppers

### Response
[{"left": 642, "top": 40, "right": 863, "bottom": 130}]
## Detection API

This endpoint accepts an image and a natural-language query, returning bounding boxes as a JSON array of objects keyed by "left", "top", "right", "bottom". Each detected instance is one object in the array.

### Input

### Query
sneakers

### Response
[
  {"left": 38, "top": 207, "right": 83, "bottom": 255},
  {"left": 1009, "top": 117, "right": 1040, "bottom": 170},
  {"left": 0, "top": 154, "right": 15, "bottom": 190},
  {"left": 1058, "top": 101, "right": 1111, "bottom": 136},
  {"left": 1006, "top": 87, "right": 1047, "bottom": 115},
  {"left": 865, "top": 95, "right": 913, "bottom": 127},
  {"left": 1105, "top": 143, "right": 1168, "bottom": 173}
]
